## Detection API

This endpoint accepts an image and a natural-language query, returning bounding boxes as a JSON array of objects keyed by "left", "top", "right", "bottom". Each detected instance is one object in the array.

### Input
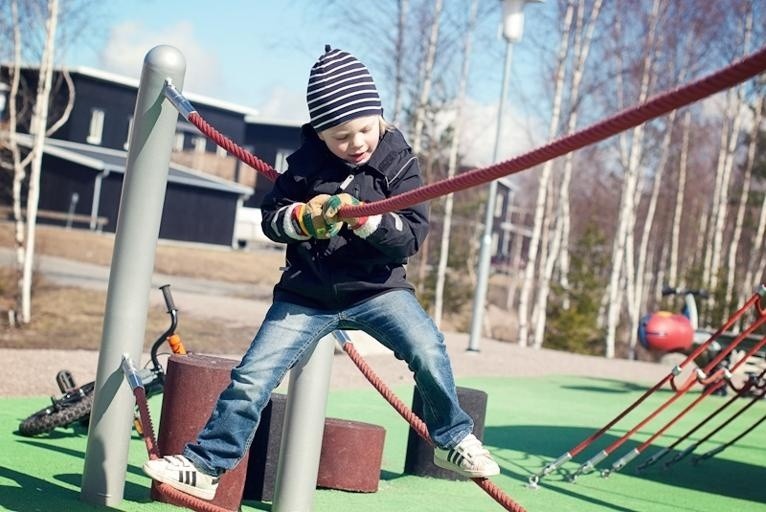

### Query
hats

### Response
[{"left": 307, "top": 44, "right": 383, "bottom": 131}]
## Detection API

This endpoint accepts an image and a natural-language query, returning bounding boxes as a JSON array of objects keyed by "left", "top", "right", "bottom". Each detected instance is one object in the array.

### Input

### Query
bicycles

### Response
[{"left": 17, "top": 283, "right": 191, "bottom": 439}]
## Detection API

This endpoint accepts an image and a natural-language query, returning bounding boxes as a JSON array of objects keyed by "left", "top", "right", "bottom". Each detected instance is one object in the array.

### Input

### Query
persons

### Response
[{"left": 142, "top": 45, "right": 499, "bottom": 500}]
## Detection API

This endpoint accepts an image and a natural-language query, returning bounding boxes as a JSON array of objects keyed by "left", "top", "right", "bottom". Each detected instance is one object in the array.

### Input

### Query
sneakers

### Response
[
  {"left": 143, "top": 455, "right": 219, "bottom": 500},
  {"left": 433, "top": 432, "right": 500, "bottom": 478}
]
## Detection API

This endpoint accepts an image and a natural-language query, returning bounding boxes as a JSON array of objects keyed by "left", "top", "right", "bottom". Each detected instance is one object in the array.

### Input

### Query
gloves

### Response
[
  {"left": 323, "top": 192, "right": 367, "bottom": 231},
  {"left": 291, "top": 194, "right": 343, "bottom": 240}
]
